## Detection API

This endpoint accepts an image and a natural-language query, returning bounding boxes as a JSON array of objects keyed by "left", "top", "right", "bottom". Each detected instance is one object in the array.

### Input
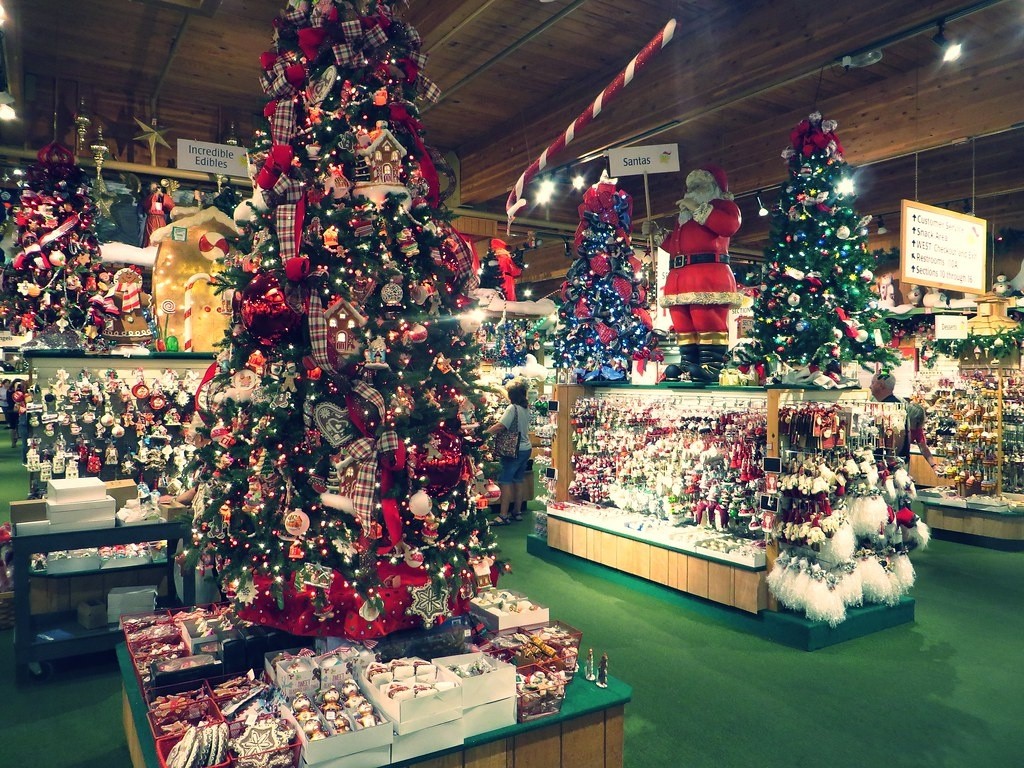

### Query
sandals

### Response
[
  {"left": 510, "top": 512, "right": 523, "bottom": 521},
  {"left": 489, "top": 515, "right": 511, "bottom": 525}
]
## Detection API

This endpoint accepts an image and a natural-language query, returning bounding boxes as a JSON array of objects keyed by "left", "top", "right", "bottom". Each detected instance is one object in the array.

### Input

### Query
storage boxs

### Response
[
  {"left": 120, "top": 588, "right": 583, "bottom": 768},
  {"left": 9, "top": 477, "right": 188, "bottom": 574},
  {"left": 107, "top": 586, "right": 160, "bottom": 624},
  {"left": 78, "top": 596, "right": 108, "bottom": 631}
]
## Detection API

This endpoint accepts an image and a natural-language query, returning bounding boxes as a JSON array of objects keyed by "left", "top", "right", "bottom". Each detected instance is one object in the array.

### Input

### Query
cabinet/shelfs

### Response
[
  {"left": 114, "top": 637, "right": 634, "bottom": 767},
  {"left": 8, "top": 520, "right": 193, "bottom": 691}
]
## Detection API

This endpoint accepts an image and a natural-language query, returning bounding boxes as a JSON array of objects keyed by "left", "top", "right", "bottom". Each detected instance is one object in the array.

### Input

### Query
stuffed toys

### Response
[
  {"left": 293, "top": 678, "right": 376, "bottom": 744},
  {"left": 993, "top": 272, "right": 1011, "bottom": 297},
  {"left": 878, "top": 272, "right": 946, "bottom": 308}
]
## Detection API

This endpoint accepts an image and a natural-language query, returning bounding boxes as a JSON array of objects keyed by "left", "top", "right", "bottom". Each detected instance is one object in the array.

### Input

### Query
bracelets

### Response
[{"left": 931, "top": 464, "right": 935, "bottom": 467}]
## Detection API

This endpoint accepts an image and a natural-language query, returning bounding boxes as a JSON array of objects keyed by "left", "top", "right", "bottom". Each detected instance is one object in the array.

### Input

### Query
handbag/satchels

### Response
[{"left": 493, "top": 404, "right": 521, "bottom": 458}]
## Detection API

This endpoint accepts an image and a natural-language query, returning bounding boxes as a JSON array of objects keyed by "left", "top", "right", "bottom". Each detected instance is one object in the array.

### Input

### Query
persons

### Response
[
  {"left": 483, "top": 377, "right": 532, "bottom": 526},
  {"left": 870, "top": 372, "right": 911, "bottom": 476},
  {"left": 159, "top": 484, "right": 198, "bottom": 506},
  {"left": 641, "top": 162, "right": 742, "bottom": 382},
  {"left": 906, "top": 403, "right": 943, "bottom": 478}
]
